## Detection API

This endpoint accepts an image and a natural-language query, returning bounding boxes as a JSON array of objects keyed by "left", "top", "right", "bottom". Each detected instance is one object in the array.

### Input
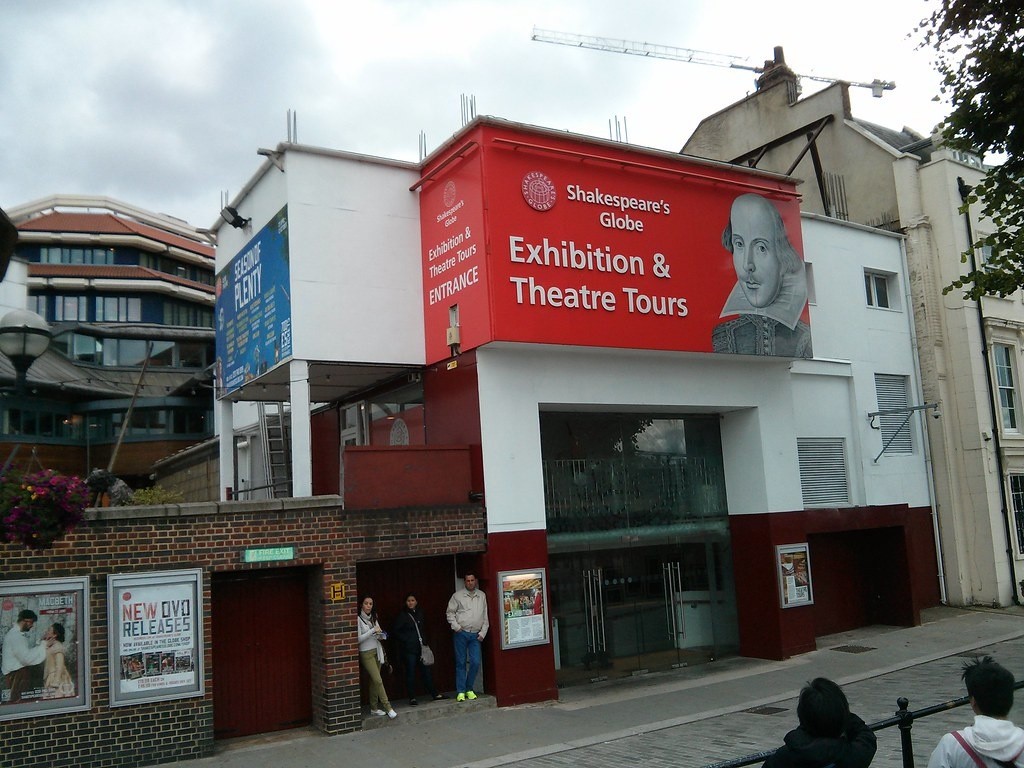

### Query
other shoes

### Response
[
  {"left": 409, "top": 697, "right": 418, "bottom": 706},
  {"left": 433, "top": 694, "right": 449, "bottom": 700}
]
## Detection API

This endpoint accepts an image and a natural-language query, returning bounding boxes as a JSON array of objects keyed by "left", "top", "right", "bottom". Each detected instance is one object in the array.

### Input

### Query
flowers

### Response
[{"left": 0, "top": 463, "right": 92, "bottom": 544}]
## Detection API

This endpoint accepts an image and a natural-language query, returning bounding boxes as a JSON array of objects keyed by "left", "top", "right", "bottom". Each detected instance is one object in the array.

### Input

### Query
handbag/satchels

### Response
[
  {"left": 420, "top": 645, "right": 434, "bottom": 666},
  {"left": 382, "top": 646, "right": 393, "bottom": 674}
]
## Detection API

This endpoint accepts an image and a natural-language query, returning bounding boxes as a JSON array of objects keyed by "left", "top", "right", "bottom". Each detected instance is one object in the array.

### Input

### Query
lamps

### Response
[{"left": 220, "top": 206, "right": 252, "bottom": 228}]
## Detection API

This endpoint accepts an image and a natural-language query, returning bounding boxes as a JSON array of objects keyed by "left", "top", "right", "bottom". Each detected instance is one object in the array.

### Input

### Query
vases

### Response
[{"left": 24, "top": 530, "right": 56, "bottom": 549}]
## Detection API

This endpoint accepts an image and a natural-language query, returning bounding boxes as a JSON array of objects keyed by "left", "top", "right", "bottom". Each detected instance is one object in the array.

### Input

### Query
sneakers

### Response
[
  {"left": 370, "top": 708, "right": 386, "bottom": 715},
  {"left": 387, "top": 709, "right": 398, "bottom": 719},
  {"left": 457, "top": 692, "right": 465, "bottom": 701},
  {"left": 466, "top": 691, "right": 477, "bottom": 700}
]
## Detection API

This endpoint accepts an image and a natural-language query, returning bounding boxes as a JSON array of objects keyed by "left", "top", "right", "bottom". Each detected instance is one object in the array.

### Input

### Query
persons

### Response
[
  {"left": 391, "top": 591, "right": 448, "bottom": 707},
  {"left": 761, "top": 677, "right": 877, "bottom": 768},
  {"left": 42, "top": 622, "right": 76, "bottom": 699},
  {"left": 927, "top": 653, "right": 1024, "bottom": 768},
  {"left": 357, "top": 593, "right": 398, "bottom": 720},
  {"left": 445, "top": 570, "right": 490, "bottom": 702},
  {"left": 1, "top": 609, "right": 58, "bottom": 702}
]
